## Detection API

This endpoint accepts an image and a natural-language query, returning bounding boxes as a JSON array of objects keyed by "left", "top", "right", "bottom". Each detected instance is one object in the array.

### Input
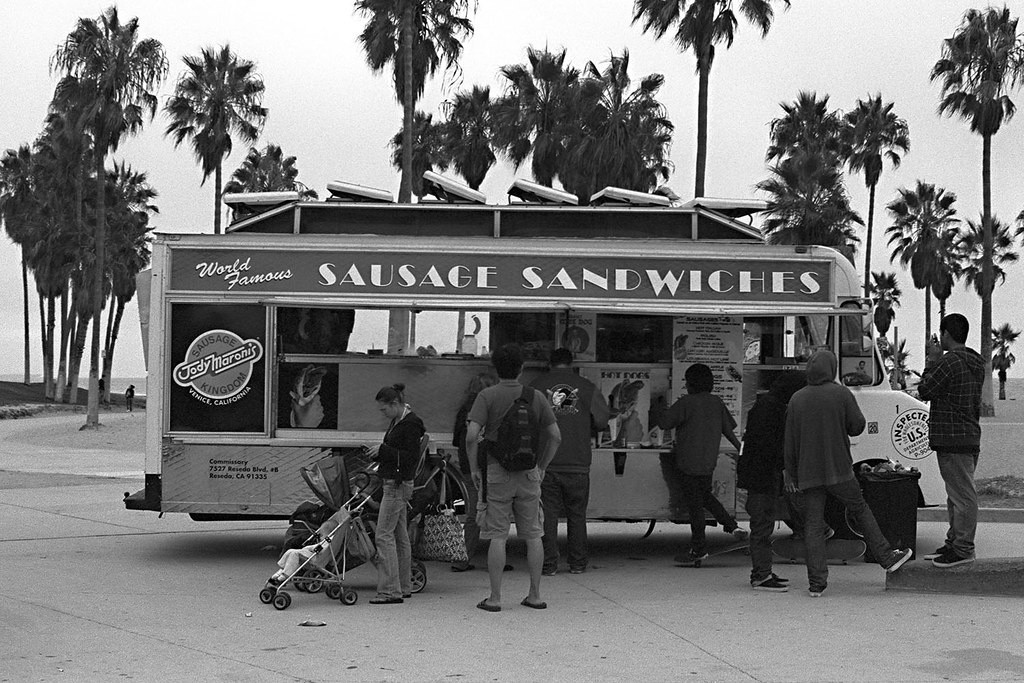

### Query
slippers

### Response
[
  {"left": 520, "top": 596, "right": 547, "bottom": 609},
  {"left": 478, "top": 598, "right": 501, "bottom": 611}
]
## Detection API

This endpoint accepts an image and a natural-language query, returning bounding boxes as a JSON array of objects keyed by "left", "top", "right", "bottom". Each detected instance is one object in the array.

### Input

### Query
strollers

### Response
[{"left": 260, "top": 453, "right": 452, "bottom": 610}]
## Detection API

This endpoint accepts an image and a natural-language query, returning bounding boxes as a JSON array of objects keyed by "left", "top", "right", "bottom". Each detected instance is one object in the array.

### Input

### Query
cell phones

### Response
[{"left": 360, "top": 444, "right": 371, "bottom": 455}]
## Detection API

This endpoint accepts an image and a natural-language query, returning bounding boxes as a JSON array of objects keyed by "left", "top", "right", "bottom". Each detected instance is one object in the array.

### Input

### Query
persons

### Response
[
  {"left": 917, "top": 314, "right": 986, "bottom": 568},
  {"left": 529, "top": 347, "right": 609, "bottom": 576},
  {"left": 291, "top": 395, "right": 324, "bottom": 428},
  {"left": 786, "top": 351, "right": 913, "bottom": 598},
  {"left": 366, "top": 383, "right": 427, "bottom": 604},
  {"left": 466, "top": 343, "right": 561, "bottom": 613},
  {"left": 271, "top": 519, "right": 339, "bottom": 584},
  {"left": 125, "top": 385, "right": 135, "bottom": 412},
  {"left": 742, "top": 370, "right": 809, "bottom": 592},
  {"left": 659, "top": 363, "right": 748, "bottom": 561},
  {"left": 99, "top": 374, "right": 106, "bottom": 404},
  {"left": 569, "top": 329, "right": 581, "bottom": 352},
  {"left": 451, "top": 373, "right": 514, "bottom": 573},
  {"left": 855, "top": 361, "right": 866, "bottom": 374}
]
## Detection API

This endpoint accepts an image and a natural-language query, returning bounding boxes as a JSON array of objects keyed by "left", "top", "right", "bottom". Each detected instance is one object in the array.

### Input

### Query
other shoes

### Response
[
  {"left": 370, "top": 595, "right": 404, "bottom": 604},
  {"left": 272, "top": 568, "right": 283, "bottom": 579},
  {"left": 403, "top": 593, "right": 412, "bottom": 598},
  {"left": 568, "top": 562, "right": 586, "bottom": 573},
  {"left": 542, "top": 563, "right": 559, "bottom": 575},
  {"left": 277, "top": 574, "right": 287, "bottom": 581},
  {"left": 824, "top": 528, "right": 835, "bottom": 539}
]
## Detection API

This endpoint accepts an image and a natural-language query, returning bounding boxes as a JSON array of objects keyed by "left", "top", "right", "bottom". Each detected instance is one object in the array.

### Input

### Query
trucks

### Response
[{"left": 120, "top": 227, "right": 941, "bottom": 562}]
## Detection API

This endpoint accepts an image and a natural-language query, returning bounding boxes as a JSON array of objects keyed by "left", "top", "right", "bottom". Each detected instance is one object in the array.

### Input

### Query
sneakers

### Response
[
  {"left": 809, "top": 582, "right": 828, "bottom": 597},
  {"left": 924, "top": 545, "right": 951, "bottom": 559},
  {"left": 751, "top": 579, "right": 789, "bottom": 592},
  {"left": 931, "top": 551, "right": 975, "bottom": 567},
  {"left": 729, "top": 527, "right": 747, "bottom": 539},
  {"left": 886, "top": 548, "right": 912, "bottom": 572},
  {"left": 770, "top": 572, "right": 789, "bottom": 585},
  {"left": 687, "top": 550, "right": 708, "bottom": 559}
]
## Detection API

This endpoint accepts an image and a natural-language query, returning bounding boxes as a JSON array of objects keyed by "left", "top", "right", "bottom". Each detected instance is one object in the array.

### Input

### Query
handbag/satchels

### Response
[
  {"left": 416, "top": 504, "right": 469, "bottom": 564},
  {"left": 345, "top": 519, "right": 375, "bottom": 562},
  {"left": 481, "top": 387, "right": 539, "bottom": 473}
]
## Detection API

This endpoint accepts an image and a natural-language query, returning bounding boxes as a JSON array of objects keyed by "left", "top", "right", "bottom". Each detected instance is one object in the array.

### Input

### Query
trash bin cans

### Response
[{"left": 858, "top": 471, "right": 922, "bottom": 563}]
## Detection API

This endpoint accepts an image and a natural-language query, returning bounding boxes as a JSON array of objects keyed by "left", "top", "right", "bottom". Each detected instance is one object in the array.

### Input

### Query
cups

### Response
[{"left": 613, "top": 452, "right": 626, "bottom": 477}]
